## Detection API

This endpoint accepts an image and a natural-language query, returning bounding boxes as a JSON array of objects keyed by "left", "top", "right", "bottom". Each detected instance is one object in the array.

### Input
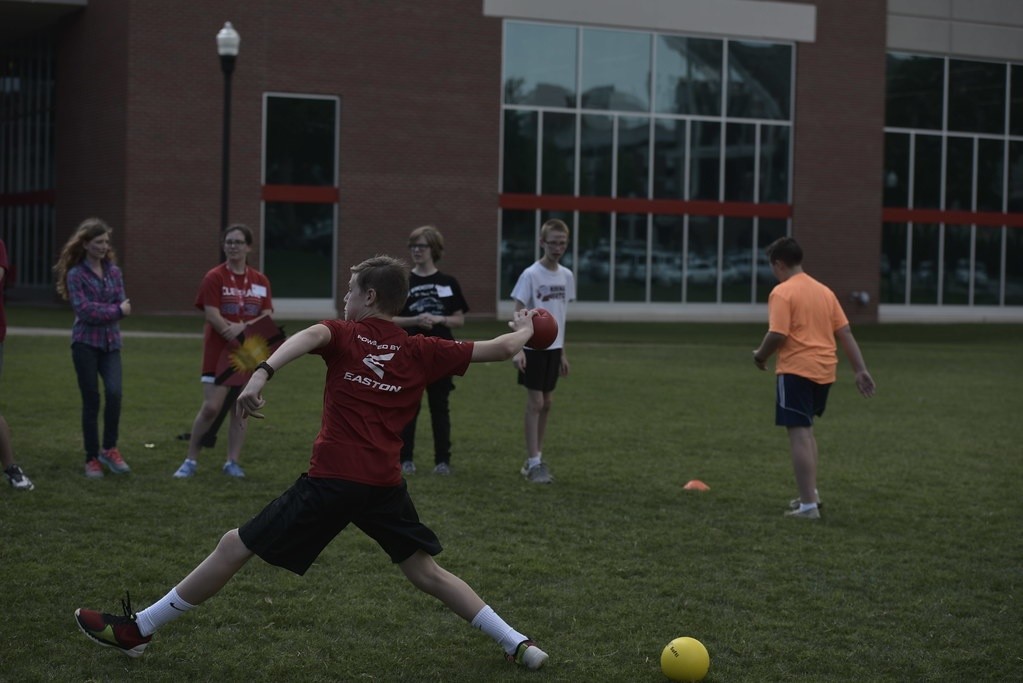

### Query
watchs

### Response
[{"left": 255, "top": 360, "right": 275, "bottom": 382}]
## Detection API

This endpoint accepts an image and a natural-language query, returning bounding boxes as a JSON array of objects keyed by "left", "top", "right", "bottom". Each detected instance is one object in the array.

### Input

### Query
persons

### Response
[
  {"left": 53, "top": 218, "right": 132, "bottom": 477},
  {"left": 0, "top": 241, "right": 35, "bottom": 492},
  {"left": 510, "top": 219, "right": 575, "bottom": 483},
  {"left": 75, "top": 256, "right": 550, "bottom": 673},
  {"left": 752, "top": 237, "right": 875, "bottom": 521},
  {"left": 400, "top": 226, "right": 470, "bottom": 477},
  {"left": 173, "top": 225, "right": 274, "bottom": 481}
]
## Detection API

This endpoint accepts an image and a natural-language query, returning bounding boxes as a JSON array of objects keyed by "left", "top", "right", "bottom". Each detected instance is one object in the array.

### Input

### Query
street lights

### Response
[{"left": 215, "top": 20, "right": 241, "bottom": 266}]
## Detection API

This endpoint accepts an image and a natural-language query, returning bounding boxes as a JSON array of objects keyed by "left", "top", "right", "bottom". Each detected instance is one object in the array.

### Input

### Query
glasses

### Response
[
  {"left": 408, "top": 243, "right": 430, "bottom": 251},
  {"left": 224, "top": 239, "right": 247, "bottom": 245},
  {"left": 543, "top": 239, "right": 567, "bottom": 248}
]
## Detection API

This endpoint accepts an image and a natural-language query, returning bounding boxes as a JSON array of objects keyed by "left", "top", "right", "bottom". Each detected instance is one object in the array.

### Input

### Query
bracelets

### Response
[
  {"left": 755, "top": 353, "right": 765, "bottom": 364},
  {"left": 118, "top": 304, "right": 122, "bottom": 318}
]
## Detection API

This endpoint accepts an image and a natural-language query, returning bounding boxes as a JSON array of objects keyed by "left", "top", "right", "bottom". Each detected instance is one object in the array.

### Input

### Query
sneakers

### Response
[
  {"left": 223, "top": 460, "right": 246, "bottom": 479},
  {"left": 784, "top": 489, "right": 822, "bottom": 519},
  {"left": 172, "top": 458, "right": 197, "bottom": 478},
  {"left": 503, "top": 636, "right": 550, "bottom": 671},
  {"left": 434, "top": 461, "right": 451, "bottom": 477},
  {"left": 74, "top": 608, "right": 155, "bottom": 659},
  {"left": 4, "top": 465, "right": 34, "bottom": 491},
  {"left": 521, "top": 457, "right": 554, "bottom": 484},
  {"left": 98, "top": 447, "right": 130, "bottom": 474},
  {"left": 84, "top": 453, "right": 104, "bottom": 479},
  {"left": 402, "top": 460, "right": 417, "bottom": 476}
]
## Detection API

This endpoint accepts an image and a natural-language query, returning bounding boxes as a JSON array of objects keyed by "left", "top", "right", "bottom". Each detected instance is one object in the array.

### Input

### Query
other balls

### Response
[
  {"left": 523, "top": 308, "right": 558, "bottom": 350},
  {"left": 661, "top": 637, "right": 709, "bottom": 681}
]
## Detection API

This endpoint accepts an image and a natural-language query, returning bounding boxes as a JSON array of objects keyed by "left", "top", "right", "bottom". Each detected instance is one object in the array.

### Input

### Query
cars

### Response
[
  {"left": 881, "top": 254, "right": 1000, "bottom": 292},
  {"left": 499, "top": 237, "right": 781, "bottom": 289}
]
